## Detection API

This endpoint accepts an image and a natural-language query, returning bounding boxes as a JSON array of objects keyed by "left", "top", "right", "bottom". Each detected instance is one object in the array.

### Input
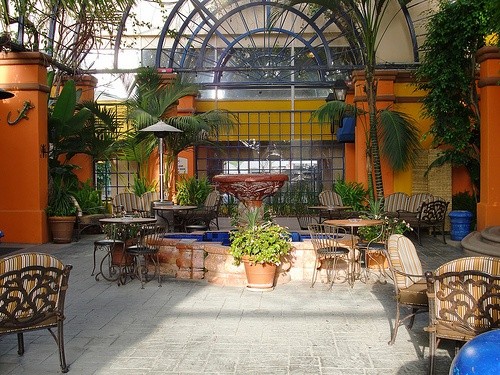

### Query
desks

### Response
[
  {"left": 153, "top": 205, "right": 198, "bottom": 234},
  {"left": 322, "top": 220, "right": 383, "bottom": 274},
  {"left": 98, "top": 217, "right": 157, "bottom": 284},
  {"left": 307, "top": 206, "right": 352, "bottom": 227}
]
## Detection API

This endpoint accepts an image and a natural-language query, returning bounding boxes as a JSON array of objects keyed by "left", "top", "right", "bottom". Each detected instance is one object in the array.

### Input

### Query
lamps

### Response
[
  {"left": 324, "top": 93, "right": 335, "bottom": 134},
  {"left": 333, "top": 78, "right": 350, "bottom": 127}
]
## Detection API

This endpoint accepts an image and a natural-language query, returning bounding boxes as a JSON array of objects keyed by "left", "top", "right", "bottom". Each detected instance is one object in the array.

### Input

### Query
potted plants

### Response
[
  {"left": 45, "top": 183, "right": 78, "bottom": 244},
  {"left": 230, "top": 214, "right": 293, "bottom": 290}
]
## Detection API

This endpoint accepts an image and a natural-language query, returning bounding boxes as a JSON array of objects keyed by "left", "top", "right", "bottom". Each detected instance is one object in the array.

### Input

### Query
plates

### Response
[
  {"left": 348, "top": 219, "right": 362, "bottom": 222},
  {"left": 172, "top": 205, "right": 181, "bottom": 208}
]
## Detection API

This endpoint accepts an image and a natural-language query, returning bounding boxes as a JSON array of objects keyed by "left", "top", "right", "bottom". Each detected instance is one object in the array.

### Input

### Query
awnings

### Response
[{"left": 336, "top": 116, "right": 356, "bottom": 144}]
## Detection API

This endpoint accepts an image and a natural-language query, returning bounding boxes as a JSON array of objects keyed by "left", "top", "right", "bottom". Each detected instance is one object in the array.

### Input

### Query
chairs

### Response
[
  {"left": 380, "top": 234, "right": 436, "bottom": 345},
  {"left": 0, "top": 252, "right": 73, "bottom": 373},
  {"left": 295, "top": 190, "right": 449, "bottom": 290},
  {"left": 91, "top": 187, "right": 223, "bottom": 288},
  {"left": 425, "top": 257, "right": 500, "bottom": 375}
]
